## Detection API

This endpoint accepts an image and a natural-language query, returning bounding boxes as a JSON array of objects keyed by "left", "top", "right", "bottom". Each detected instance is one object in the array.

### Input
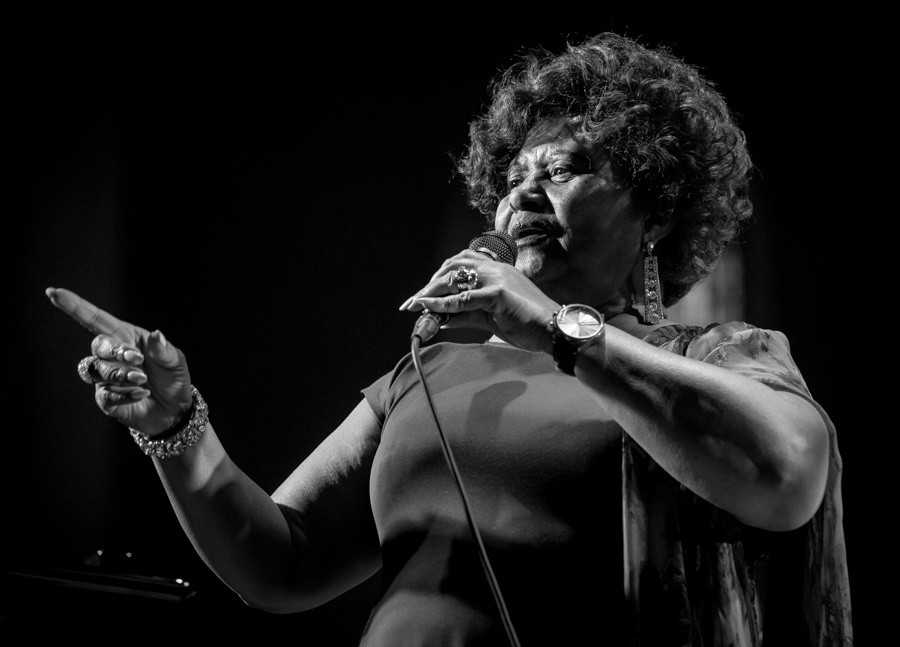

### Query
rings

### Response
[{"left": 78, "top": 355, "right": 104, "bottom": 385}]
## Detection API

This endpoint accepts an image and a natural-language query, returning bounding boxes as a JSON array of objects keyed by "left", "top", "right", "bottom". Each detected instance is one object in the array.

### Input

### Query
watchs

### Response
[{"left": 550, "top": 303, "right": 605, "bottom": 377}]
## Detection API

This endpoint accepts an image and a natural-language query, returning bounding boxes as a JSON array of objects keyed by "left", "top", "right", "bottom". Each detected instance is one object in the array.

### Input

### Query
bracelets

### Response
[{"left": 128, "top": 384, "right": 208, "bottom": 459}]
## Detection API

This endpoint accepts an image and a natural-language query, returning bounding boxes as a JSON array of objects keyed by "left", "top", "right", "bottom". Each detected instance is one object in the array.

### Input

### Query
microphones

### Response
[{"left": 410, "top": 229, "right": 518, "bottom": 344}]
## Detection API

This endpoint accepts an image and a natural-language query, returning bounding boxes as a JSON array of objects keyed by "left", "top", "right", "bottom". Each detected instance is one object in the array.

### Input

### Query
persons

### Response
[{"left": 46, "top": 33, "right": 852, "bottom": 647}]
